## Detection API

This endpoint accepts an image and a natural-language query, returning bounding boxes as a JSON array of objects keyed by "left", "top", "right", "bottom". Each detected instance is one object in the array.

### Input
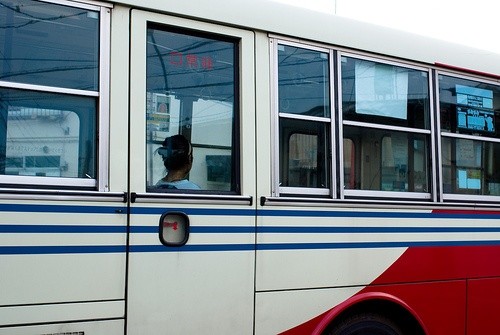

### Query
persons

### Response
[{"left": 152, "top": 134, "right": 202, "bottom": 190}]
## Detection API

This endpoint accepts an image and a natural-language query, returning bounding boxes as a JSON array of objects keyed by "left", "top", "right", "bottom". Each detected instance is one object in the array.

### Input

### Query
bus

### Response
[{"left": 0, "top": 0, "right": 498, "bottom": 331}]
